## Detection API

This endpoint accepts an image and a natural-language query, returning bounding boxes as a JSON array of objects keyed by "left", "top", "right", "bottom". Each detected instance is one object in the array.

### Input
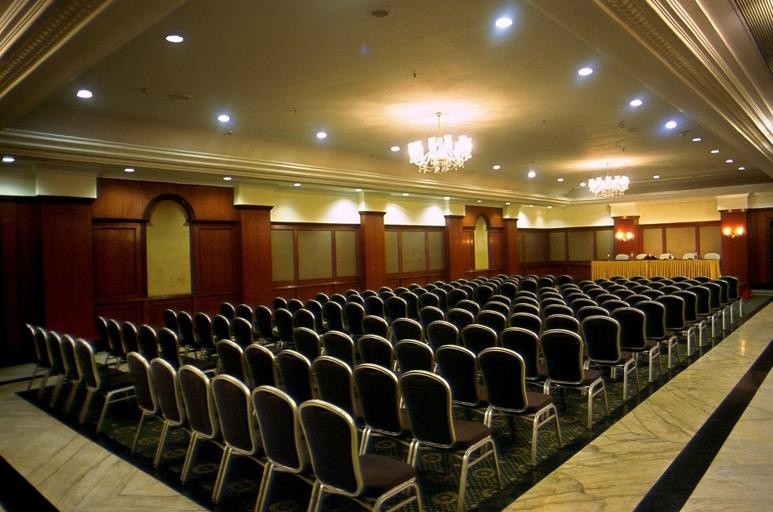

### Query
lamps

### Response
[
  {"left": 723, "top": 227, "right": 744, "bottom": 239},
  {"left": 407, "top": 112, "right": 474, "bottom": 175},
  {"left": 587, "top": 164, "right": 631, "bottom": 198},
  {"left": 615, "top": 231, "right": 633, "bottom": 242}
]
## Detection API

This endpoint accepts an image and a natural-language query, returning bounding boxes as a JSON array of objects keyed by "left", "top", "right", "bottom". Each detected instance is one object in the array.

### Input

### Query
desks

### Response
[{"left": 591, "top": 259, "right": 721, "bottom": 281}]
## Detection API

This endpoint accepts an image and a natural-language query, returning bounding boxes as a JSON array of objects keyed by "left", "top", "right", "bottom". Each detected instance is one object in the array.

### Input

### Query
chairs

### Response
[{"left": 615, "top": 252, "right": 721, "bottom": 260}]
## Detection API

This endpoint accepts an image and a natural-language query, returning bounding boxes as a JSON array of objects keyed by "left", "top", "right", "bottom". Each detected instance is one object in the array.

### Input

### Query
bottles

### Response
[
  {"left": 693, "top": 249, "right": 698, "bottom": 261},
  {"left": 607, "top": 251, "right": 611, "bottom": 261},
  {"left": 630, "top": 250, "right": 633, "bottom": 261},
  {"left": 668, "top": 249, "right": 672, "bottom": 260},
  {"left": 647, "top": 249, "right": 651, "bottom": 260}
]
